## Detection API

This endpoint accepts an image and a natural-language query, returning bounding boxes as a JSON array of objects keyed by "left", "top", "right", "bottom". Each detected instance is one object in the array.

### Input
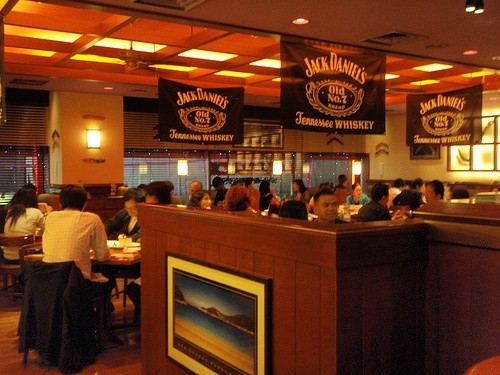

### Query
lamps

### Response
[{"left": 86, "top": 129, "right": 102, "bottom": 149}]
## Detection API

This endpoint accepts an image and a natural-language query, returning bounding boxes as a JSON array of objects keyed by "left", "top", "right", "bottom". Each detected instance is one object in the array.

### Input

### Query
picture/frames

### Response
[
  {"left": 410, "top": 145, "right": 440, "bottom": 160},
  {"left": 165, "top": 252, "right": 274, "bottom": 375}
]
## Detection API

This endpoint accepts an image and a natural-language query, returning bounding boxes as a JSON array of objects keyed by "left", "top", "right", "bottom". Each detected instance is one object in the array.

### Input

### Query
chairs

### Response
[{"left": 0, "top": 204, "right": 134, "bottom": 375}]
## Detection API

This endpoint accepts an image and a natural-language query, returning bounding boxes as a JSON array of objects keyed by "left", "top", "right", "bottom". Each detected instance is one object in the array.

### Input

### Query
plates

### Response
[
  {"left": 132, "top": 242, "right": 140, "bottom": 246},
  {"left": 123, "top": 247, "right": 140, "bottom": 253},
  {"left": 107, "top": 240, "right": 123, "bottom": 248}
]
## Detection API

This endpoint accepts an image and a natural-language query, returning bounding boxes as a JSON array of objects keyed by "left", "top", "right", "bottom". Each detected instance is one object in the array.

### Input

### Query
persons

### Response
[
  {"left": 210, "top": 177, "right": 227, "bottom": 206},
  {"left": 347, "top": 183, "right": 368, "bottom": 205},
  {"left": 417, "top": 180, "right": 469, "bottom": 212},
  {"left": 189, "top": 181, "right": 201, "bottom": 200},
  {"left": 186, "top": 189, "right": 211, "bottom": 209},
  {"left": 312, "top": 188, "right": 347, "bottom": 224},
  {"left": 280, "top": 199, "right": 307, "bottom": 220},
  {"left": 285, "top": 179, "right": 310, "bottom": 203},
  {"left": 309, "top": 184, "right": 327, "bottom": 206},
  {"left": 387, "top": 178, "right": 405, "bottom": 208},
  {"left": 225, "top": 185, "right": 253, "bottom": 212},
  {"left": 245, "top": 178, "right": 258, "bottom": 192},
  {"left": 103, "top": 188, "right": 143, "bottom": 241},
  {"left": 43, "top": 184, "right": 114, "bottom": 328},
  {"left": 259, "top": 181, "right": 275, "bottom": 210},
  {"left": 127, "top": 180, "right": 170, "bottom": 321},
  {"left": 4, "top": 187, "right": 46, "bottom": 265},
  {"left": 357, "top": 183, "right": 392, "bottom": 222},
  {"left": 333, "top": 174, "right": 347, "bottom": 191},
  {"left": 393, "top": 179, "right": 425, "bottom": 210}
]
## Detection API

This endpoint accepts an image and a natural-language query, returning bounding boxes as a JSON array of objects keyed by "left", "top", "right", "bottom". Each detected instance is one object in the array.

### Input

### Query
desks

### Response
[{"left": 91, "top": 248, "right": 140, "bottom": 277}]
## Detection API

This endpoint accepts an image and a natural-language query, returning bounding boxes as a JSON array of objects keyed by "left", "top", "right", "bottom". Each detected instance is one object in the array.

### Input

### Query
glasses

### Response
[{"left": 125, "top": 207, "right": 136, "bottom": 211}]
